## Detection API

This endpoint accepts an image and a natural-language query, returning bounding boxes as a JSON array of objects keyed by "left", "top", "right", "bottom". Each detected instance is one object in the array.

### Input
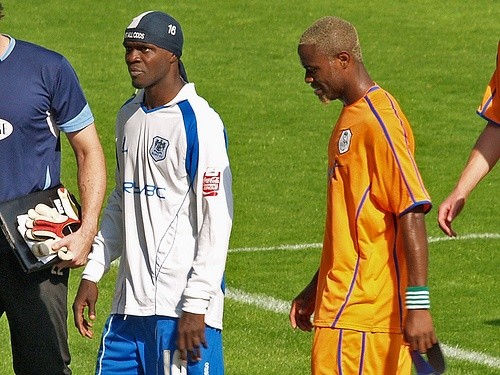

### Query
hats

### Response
[{"left": 123, "top": 10, "right": 190, "bottom": 84}]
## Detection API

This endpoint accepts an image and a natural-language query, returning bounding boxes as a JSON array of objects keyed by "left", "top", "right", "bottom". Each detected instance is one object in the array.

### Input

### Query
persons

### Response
[
  {"left": 0, "top": 4, "right": 107, "bottom": 375},
  {"left": 288, "top": 16, "right": 437, "bottom": 375},
  {"left": 437, "top": 40, "right": 500, "bottom": 237},
  {"left": 73, "top": 12, "right": 235, "bottom": 375}
]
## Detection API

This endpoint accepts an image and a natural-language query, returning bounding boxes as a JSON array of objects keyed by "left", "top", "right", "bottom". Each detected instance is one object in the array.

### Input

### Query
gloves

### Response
[{"left": 24, "top": 202, "right": 83, "bottom": 261}]
